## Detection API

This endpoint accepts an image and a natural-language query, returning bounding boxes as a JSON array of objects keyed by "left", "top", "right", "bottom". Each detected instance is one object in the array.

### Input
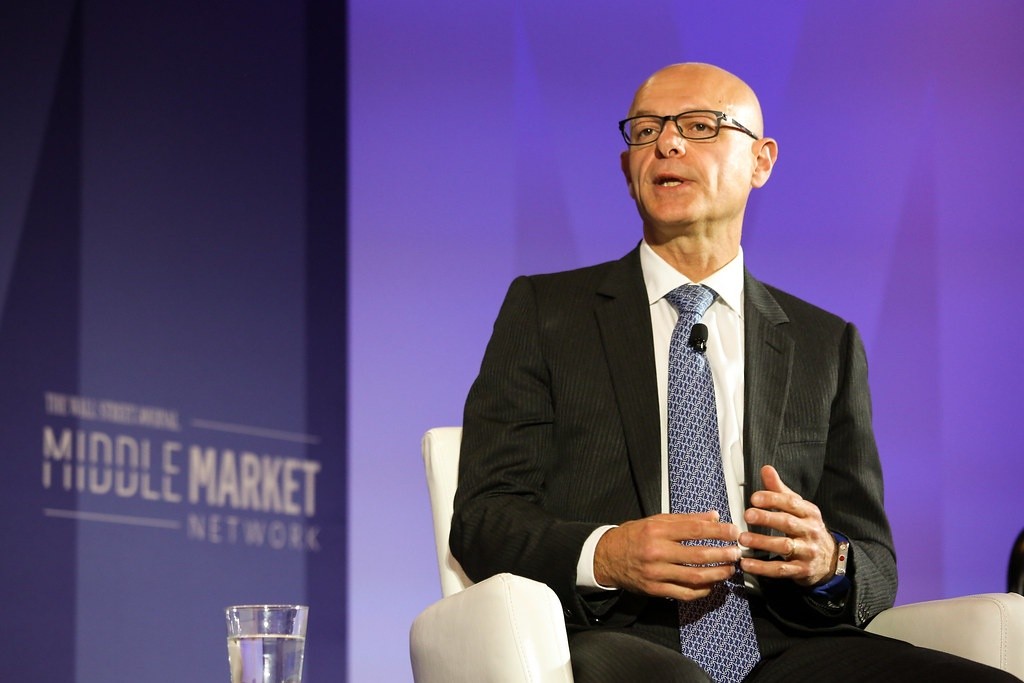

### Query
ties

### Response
[{"left": 662, "top": 285, "right": 761, "bottom": 683}]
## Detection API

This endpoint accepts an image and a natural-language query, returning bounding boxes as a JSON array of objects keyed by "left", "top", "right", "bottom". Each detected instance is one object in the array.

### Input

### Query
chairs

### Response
[{"left": 409, "top": 426, "right": 1024, "bottom": 683}]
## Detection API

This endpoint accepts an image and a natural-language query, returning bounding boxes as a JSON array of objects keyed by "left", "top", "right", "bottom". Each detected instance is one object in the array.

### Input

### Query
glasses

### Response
[{"left": 617, "top": 110, "right": 759, "bottom": 145}]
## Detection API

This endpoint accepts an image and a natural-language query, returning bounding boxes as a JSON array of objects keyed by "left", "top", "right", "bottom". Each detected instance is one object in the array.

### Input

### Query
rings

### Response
[{"left": 781, "top": 539, "right": 796, "bottom": 558}]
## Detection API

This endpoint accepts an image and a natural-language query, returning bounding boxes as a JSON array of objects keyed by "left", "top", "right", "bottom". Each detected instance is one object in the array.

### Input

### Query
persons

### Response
[{"left": 449, "top": 62, "right": 1024, "bottom": 682}]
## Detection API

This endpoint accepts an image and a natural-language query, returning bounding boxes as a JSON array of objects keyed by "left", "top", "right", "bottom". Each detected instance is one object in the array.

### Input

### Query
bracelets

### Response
[{"left": 811, "top": 532, "right": 850, "bottom": 595}]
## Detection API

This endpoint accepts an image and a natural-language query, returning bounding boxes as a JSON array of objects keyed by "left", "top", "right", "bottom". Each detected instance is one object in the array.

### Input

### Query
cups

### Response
[{"left": 224, "top": 604, "right": 310, "bottom": 683}]
